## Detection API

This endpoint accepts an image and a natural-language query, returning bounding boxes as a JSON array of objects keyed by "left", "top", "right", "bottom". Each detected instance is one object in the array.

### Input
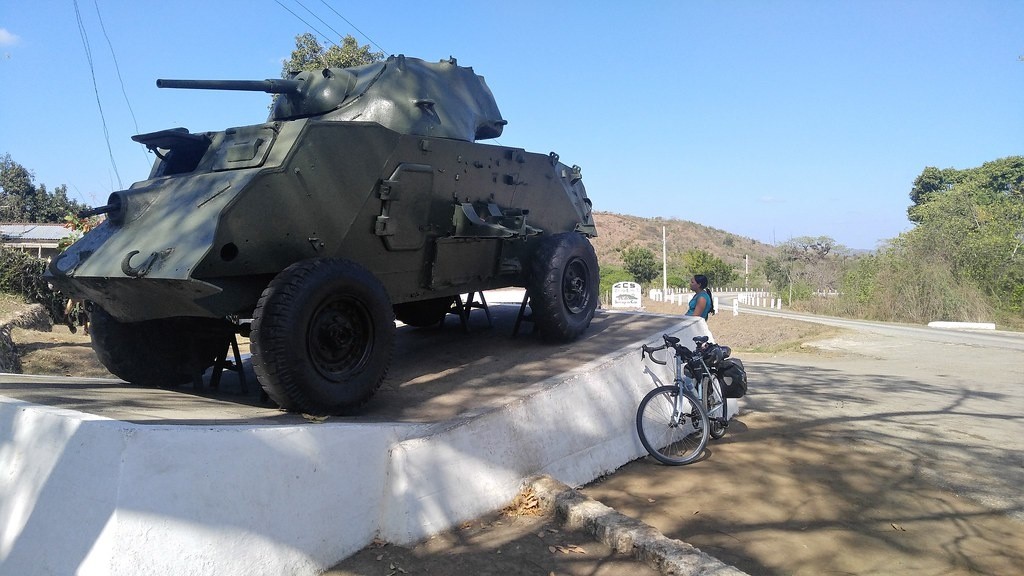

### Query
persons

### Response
[{"left": 685, "top": 275, "right": 715, "bottom": 322}]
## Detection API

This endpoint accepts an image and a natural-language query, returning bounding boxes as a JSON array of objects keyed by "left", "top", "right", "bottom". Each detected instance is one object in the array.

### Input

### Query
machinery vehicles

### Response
[{"left": 38, "top": 53, "right": 604, "bottom": 420}]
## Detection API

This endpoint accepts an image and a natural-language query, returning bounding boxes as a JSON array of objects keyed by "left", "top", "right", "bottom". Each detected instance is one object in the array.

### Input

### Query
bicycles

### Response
[{"left": 636, "top": 333, "right": 729, "bottom": 465}]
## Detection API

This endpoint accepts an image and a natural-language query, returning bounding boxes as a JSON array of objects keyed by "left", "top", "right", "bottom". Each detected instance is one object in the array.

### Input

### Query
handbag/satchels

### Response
[{"left": 718, "top": 358, "right": 747, "bottom": 398}]
